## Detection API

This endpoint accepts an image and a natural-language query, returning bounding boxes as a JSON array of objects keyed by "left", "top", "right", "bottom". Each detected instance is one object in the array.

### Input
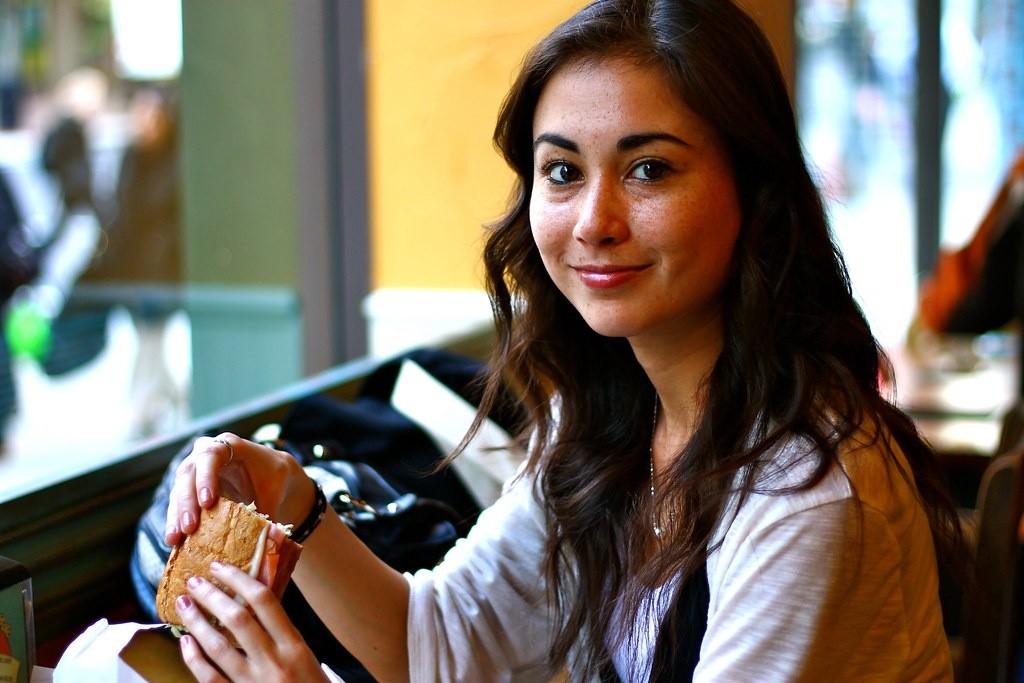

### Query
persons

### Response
[{"left": 167, "top": 0, "right": 954, "bottom": 683}]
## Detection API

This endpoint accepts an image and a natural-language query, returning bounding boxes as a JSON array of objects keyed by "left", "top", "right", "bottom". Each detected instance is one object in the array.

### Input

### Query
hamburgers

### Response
[{"left": 155, "top": 494, "right": 304, "bottom": 649}]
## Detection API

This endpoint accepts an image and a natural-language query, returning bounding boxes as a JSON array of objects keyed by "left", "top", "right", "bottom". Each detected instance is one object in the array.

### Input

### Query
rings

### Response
[{"left": 213, "top": 439, "right": 234, "bottom": 466}]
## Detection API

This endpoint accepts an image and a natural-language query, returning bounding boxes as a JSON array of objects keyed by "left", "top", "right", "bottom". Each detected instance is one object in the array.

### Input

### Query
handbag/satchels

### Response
[{"left": 131, "top": 424, "right": 456, "bottom": 624}]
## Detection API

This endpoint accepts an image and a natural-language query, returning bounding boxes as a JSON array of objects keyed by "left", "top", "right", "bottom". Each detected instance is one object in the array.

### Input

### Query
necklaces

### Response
[{"left": 649, "top": 390, "right": 678, "bottom": 538}]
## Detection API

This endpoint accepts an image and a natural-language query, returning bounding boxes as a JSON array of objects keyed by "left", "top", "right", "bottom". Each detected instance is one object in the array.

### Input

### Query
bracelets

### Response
[{"left": 289, "top": 480, "right": 327, "bottom": 544}]
